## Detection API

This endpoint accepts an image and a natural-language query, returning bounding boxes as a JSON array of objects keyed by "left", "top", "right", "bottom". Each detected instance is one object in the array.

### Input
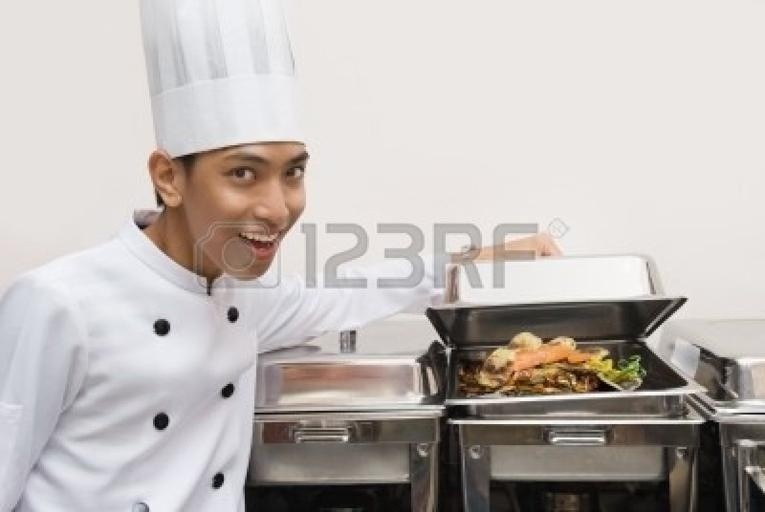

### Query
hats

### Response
[{"left": 138, "top": 0, "right": 308, "bottom": 160}]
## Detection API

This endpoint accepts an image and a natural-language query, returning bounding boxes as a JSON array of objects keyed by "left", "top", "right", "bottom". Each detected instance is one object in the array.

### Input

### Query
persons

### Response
[{"left": 0, "top": 142, "right": 563, "bottom": 512}]
